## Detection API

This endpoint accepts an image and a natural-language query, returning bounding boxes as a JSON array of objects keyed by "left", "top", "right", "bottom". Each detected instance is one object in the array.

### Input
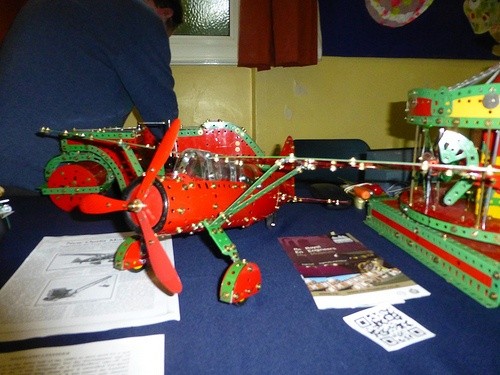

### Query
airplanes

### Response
[{"left": 40, "top": 119, "right": 500, "bottom": 306}]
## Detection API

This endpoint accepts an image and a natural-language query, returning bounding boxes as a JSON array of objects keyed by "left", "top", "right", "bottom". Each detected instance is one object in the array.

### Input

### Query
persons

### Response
[{"left": 0, "top": 0, "right": 187, "bottom": 191}]
